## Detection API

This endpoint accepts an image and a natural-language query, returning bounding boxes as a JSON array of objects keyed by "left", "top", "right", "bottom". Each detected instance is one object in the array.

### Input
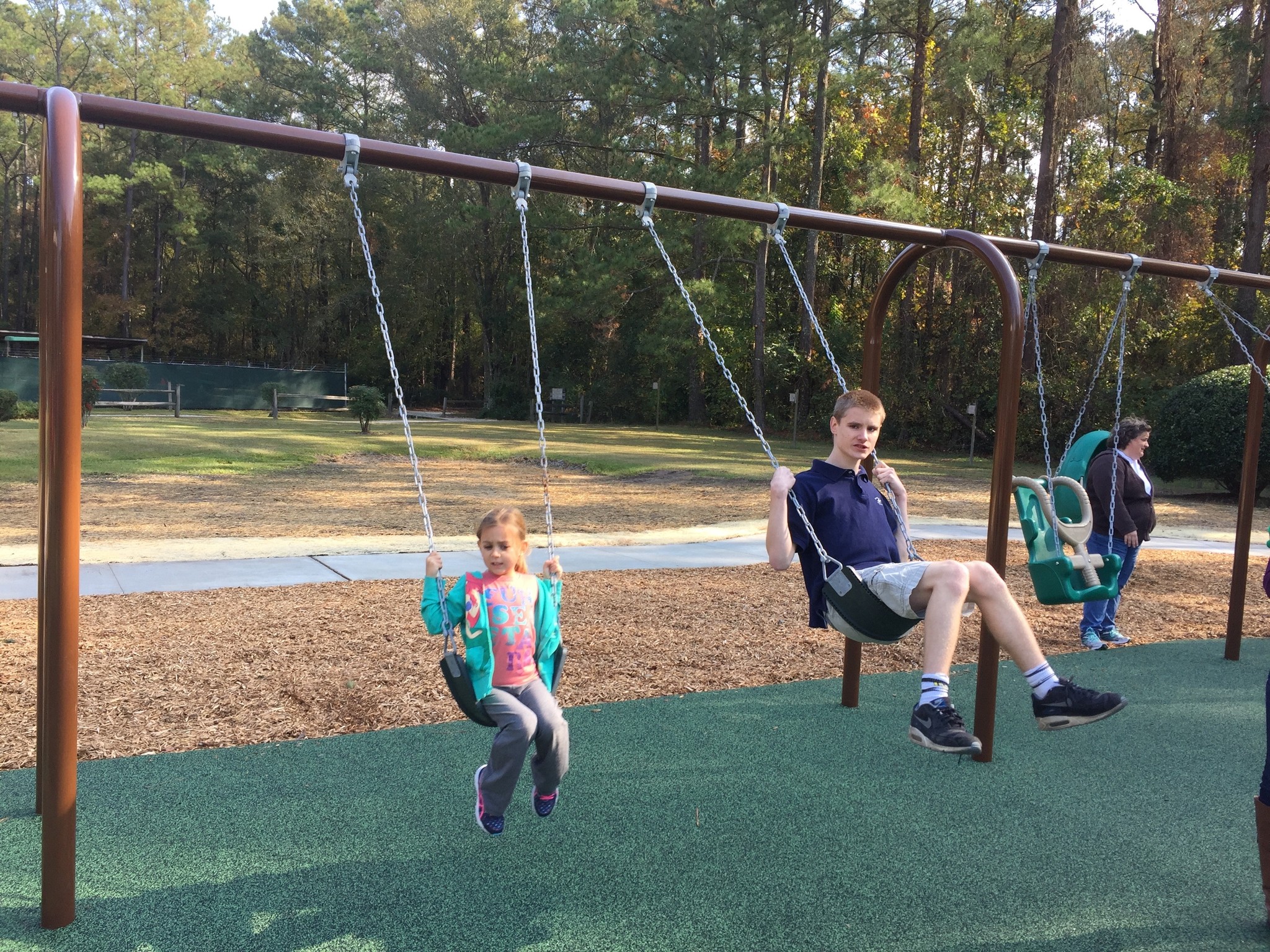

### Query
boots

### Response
[{"left": 1254, "top": 796, "right": 1269, "bottom": 927}]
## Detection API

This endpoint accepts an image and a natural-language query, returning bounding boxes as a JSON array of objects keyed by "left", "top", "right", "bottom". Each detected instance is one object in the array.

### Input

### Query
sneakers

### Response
[
  {"left": 1083, "top": 627, "right": 1109, "bottom": 650},
  {"left": 1030, "top": 676, "right": 1126, "bottom": 731},
  {"left": 908, "top": 696, "right": 982, "bottom": 765},
  {"left": 531, "top": 786, "right": 559, "bottom": 817},
  {"left": 1100, "top": 629, "right": 1130, "bottom": 644},
  {"left": 475, "top": 764, "right": 505, "bottom": 835}
]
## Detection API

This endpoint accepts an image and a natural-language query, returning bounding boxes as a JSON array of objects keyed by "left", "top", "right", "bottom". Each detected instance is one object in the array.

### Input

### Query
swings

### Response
[
  {"left": 351, "top": 178, "right": 569, "bottom": 727},
  {"left": 1010, "top": 279, "right": 1132, "bottom": 606},
  {"left": 1205, "top": 290, "right": 1270, "bottom": 549},
  {"left": 646, "top": 224, "right": 927, "bottom": 642}
]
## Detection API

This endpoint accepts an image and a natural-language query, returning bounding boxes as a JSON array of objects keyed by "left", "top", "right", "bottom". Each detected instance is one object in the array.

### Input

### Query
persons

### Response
[
  {"left": 766, "top": 389, "right": 1128, "bottom": 754},
  {"left": 422, "top": 506, "right": 572, "bottom": 836},
  {"left": 1079, "top": 418, "right": 1157, "bottom": 650},
  {"left": 1251, "top": 528, "right": 1270, "bottom": 940}
]
